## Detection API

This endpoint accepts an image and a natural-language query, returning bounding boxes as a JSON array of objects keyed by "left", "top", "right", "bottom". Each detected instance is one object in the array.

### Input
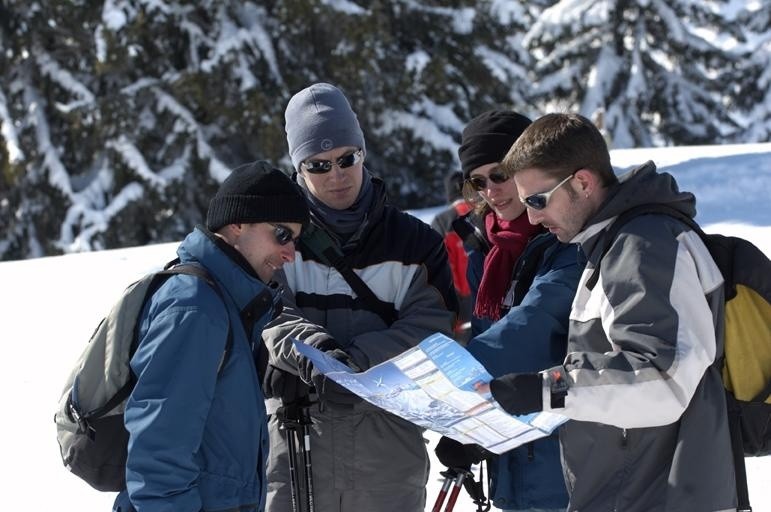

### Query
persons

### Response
[
  {"left": 474, "top": 112, "right": 754, "bottom": 512},
  {"left": 431, "top": 108, "right": 587, "bottom": 512},
  {"left": 251, "top": 82, "right": 459, "bottom": 512},
  {"left": 112, "top": 158, "right": 310, "bottom": 512},
  {"left": 430, "top": 196, "right": 485, "bottom": 331}
]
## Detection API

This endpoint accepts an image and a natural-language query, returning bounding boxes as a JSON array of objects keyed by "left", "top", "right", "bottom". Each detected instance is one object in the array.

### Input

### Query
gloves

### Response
[
  {"left": 307, "top": 338, "right": 372, "bottom": 411},
  {"left": 489, "top": 370, "right": 545, "bottom": 417}
]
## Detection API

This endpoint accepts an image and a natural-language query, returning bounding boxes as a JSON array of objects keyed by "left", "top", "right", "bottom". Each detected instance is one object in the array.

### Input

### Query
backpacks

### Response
[
  {"left": 707, "top": 230, "right": 771, "bottom": 458},
  {"left": 52, "top": 266, "right": 155, "bottom": 493}
]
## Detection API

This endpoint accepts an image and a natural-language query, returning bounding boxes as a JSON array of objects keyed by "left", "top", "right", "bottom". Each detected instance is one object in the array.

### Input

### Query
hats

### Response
[
  {"left": 284, "top": 81, "right": 368, "bottom": 174},
  {"left": 205, "top": 160, "right": 312, "bottom": 230},
  {"left": 459, "top": 109, "right": 536, "bottom": 178}
]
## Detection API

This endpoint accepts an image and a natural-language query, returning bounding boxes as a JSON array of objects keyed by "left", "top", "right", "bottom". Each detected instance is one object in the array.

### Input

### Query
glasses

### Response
[
  {"left": 520, "top": 174, "right": 574, "bottom": 210},
  {"left": 467, "top": 167, "right": 515, "bottom": 192},
  {"left": 261, "top": 219, "right": 303, "bottom": 246},
  {"left": 298, "top": 148, "right": 364, "bottom": 174}
]
